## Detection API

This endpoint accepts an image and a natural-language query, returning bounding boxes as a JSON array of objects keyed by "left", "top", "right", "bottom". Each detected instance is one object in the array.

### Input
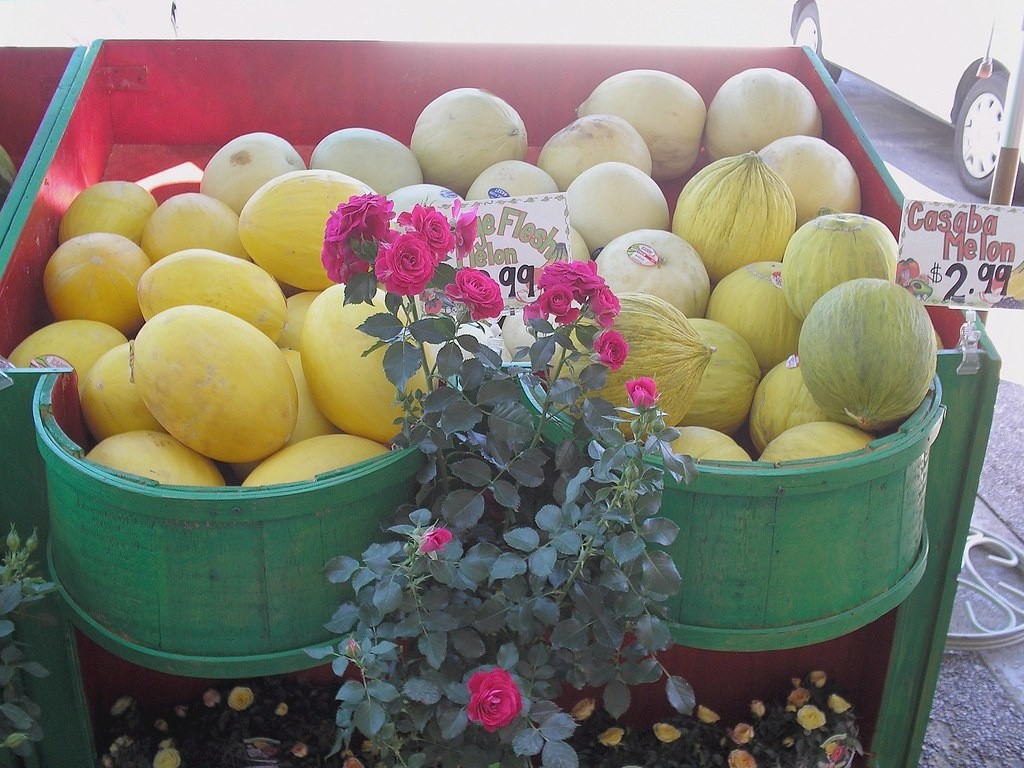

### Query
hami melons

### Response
[{"left": 7, "top": 67, "right": 938, "bottom": 487}]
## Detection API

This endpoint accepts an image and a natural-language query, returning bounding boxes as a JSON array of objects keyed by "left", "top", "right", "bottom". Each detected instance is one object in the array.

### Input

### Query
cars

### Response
[{"left": 791, "top": 0, "right": 1024, "bottom": 197}]
